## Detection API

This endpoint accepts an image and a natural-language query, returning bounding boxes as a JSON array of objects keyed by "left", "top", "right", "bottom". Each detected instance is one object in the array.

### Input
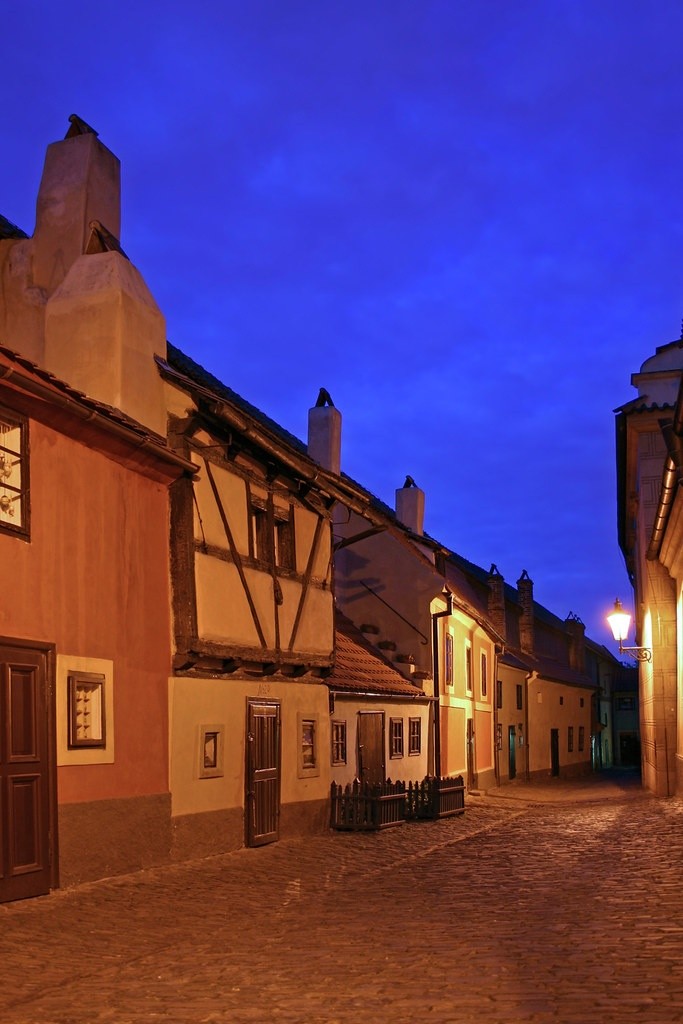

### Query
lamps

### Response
[{"left": 606, "top": 597, "right": 652, "bottom": 663}]
